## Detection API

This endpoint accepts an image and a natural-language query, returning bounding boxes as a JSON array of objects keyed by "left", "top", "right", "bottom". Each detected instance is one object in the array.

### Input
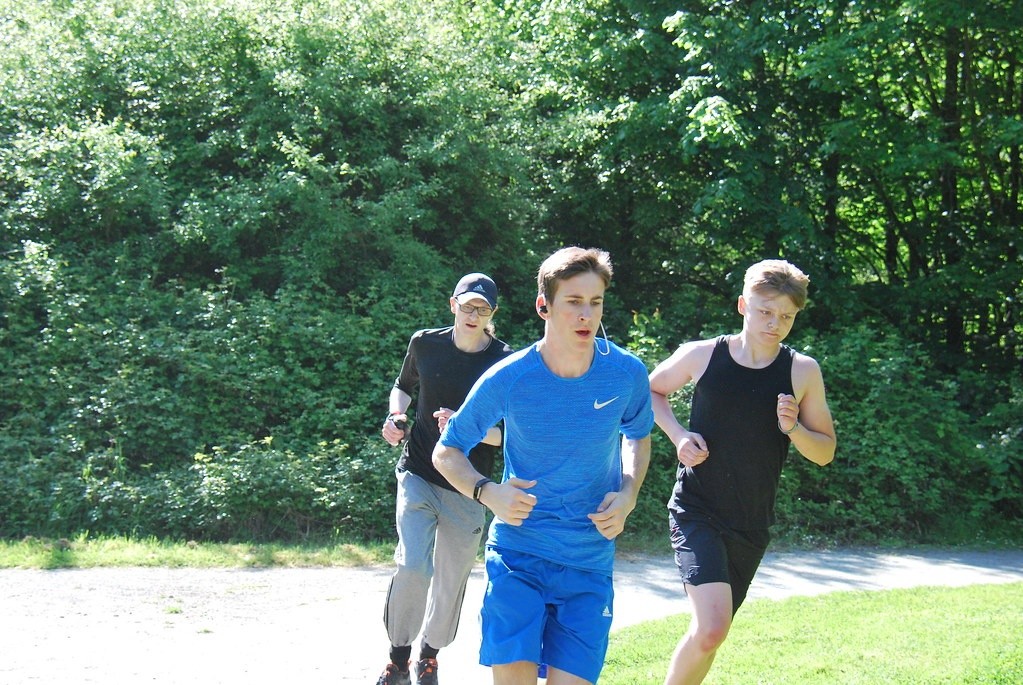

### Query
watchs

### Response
[{"left": 474, "top": 478, "right": 491, "bottom": 506}]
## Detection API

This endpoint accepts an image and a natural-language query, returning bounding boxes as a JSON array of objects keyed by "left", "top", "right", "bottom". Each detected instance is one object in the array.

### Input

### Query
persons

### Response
[
  {"left": 646, "top": 260, "right": 835, "bottom": 685},
  {"left": 432, "top": 246, "right": 655, "bottom": 685},
  {"left": 376, "top": 273, "right": 515, "bottom": 685}
]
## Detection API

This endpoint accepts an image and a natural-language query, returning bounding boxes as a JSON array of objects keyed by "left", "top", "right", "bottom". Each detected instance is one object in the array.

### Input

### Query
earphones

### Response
[{"left": 539, "top": 306, "right": 548, "bottom": 314}]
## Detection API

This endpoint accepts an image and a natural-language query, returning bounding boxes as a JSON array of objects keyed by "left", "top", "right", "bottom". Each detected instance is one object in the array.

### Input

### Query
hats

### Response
[{"left": 452, "top": 272, "right": 498, "bottom": 312}]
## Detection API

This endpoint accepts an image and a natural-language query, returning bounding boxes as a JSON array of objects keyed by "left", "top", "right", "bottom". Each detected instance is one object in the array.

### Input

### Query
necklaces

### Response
[{"left": 452, "top": 328, "right": 491, "bottom": 351}]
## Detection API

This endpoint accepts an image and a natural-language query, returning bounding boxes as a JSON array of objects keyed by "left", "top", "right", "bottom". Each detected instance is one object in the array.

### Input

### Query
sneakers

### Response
[
  {"left": 414, "top": 658, "right": 439, "bottom": 685},
  {"left": 375, "top": 660, "right": 412, "bottom": 685}
]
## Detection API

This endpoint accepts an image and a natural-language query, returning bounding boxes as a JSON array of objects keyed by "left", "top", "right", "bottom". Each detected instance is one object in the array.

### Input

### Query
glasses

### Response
[{"left": 455, "top": 299, "right": 495, "bottom": 317}]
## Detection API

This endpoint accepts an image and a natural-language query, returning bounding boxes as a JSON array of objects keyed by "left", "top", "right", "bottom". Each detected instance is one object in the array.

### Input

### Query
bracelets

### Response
[
  {"left": 778, "top": 419, "right": 798, "bottom": 434},
  {"left": 386, "top": 411, "right": 402, "bottom": 420}
]
